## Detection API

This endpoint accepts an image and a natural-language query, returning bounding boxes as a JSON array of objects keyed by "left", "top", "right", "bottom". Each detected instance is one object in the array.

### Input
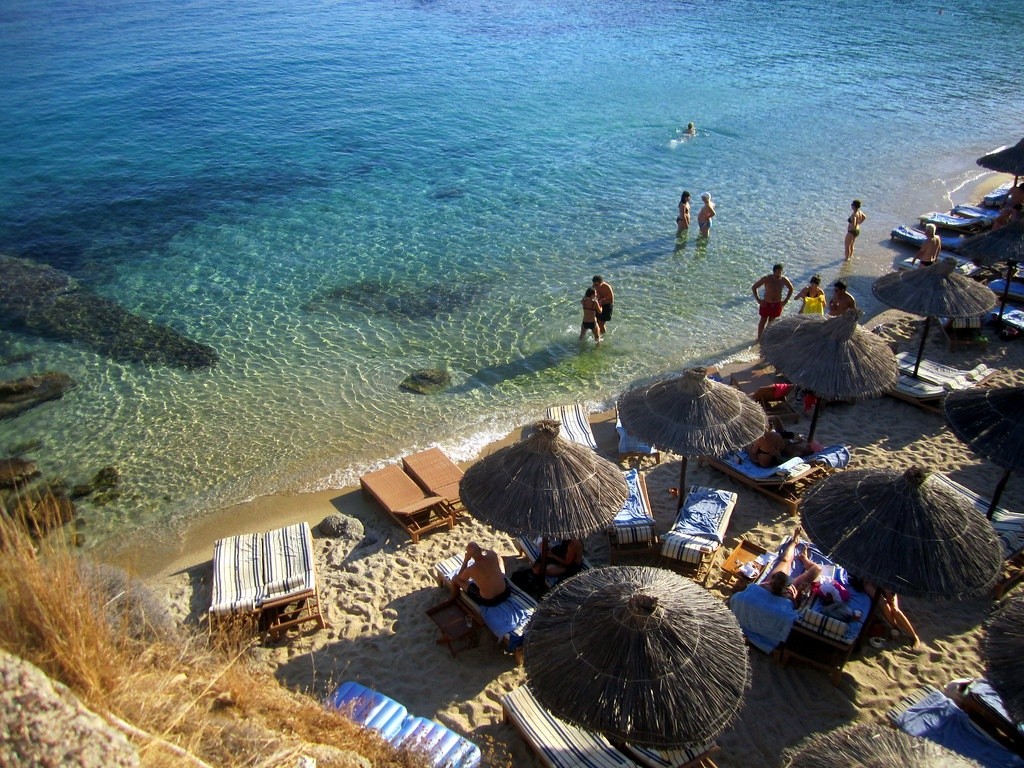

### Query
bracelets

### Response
[{"left": 785, "top": 299, "right": 789, "bottom": 302}]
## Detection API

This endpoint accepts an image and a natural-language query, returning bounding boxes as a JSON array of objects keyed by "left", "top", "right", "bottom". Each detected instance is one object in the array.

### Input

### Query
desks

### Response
[
  {"left": 427, "top": 602, "right": 478, "bottom": 658},
  {"left": 721, "top": 539, "right": 774, "bottom": 585}
]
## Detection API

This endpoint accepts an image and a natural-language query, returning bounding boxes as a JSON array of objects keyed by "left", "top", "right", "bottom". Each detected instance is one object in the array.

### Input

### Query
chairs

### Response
[
  {"left": 499, "top": 682, "right": 634, "bottom": 768},
  {"left": 544, "top": 403, "right": 598, "bottom": 452},
  {"left": 615, "top": 401, "right": 660, "bottom": 464},
  {"left": 212, "top": 533, "right": 264, "bottom": 648},
  {"left": 625, "top": 740, "right": 721, "bottom": 768},
  {"left": 437, "top": 552, "right": 537, "bottom": 648},
  {"left": 887, "top": 674, "right": 1024, "bottom": 768},
  {"left": 358, "top": 464, "right": 453, "bottom": 540},
  {"left": 658, "top": 186, "right": 1024, "bottom": 688},
  {"left": 610, "top": 469, "right": 658, "bottom": 555},
  {"left": 266, "top": 523, "right": 325, "bottom": 643},
  {"left": 517, "top": 535, "right": 592, "bottom": 588},
  {"left": 402, "top": 447, "right": 472, "bottom": 525}
]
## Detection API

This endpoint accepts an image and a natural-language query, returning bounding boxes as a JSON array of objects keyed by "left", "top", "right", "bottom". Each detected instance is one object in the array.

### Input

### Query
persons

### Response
[
  {"left": 758, "top": 524, "right": 822, "bottom": 610},
  {"left": 449, "top": 542, "right": 511, "bottom": 606},
  {"left": 992, "top": 202, "right": 1024, "bottom": 231},
  {"left": 683, "top": 123, "right": 695, "bottom": 134},
  {"left": 911, "top": 223, "right": 942, "bottom": 268},
  {"left": 580, "top": 287, "right": 602, "bottom": 339},
  {"left": 752, "top": 263, "right": 794, "bottom": 341},
  {"left": 591, "top": 275, "right": 614, "bottom": 332},
  {"left": 676, "top": 191, "right": 690, "bottom": 229},
  {"left": 532, "top": 533, "right": 584, "bottom": 576},
  {"left": 698, "top": 192, "right": 716, "bottom": 236},
  {"left": 794, "top": 275, "right": 827, "bottom": 315},
  {"left": 1001, "top": 183, "right": 1024, "bottom": 209},
  {"left": 749, "top": 422, "right": 786, "bottom": 468},
  {"left": 861, "top": 577, "right": 920, "bottom": 650},
  {"left": 828, "top": 280, "right": 855, "bottom": 316},
  {"left": 843, "top": 200, "right": 867, "bottom": 260}
]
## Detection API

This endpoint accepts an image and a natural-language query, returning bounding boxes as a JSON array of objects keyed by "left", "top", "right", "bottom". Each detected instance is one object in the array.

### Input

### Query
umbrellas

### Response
[{"left": 461, "top": 138, "right": 1023, "bottom": 768}]
